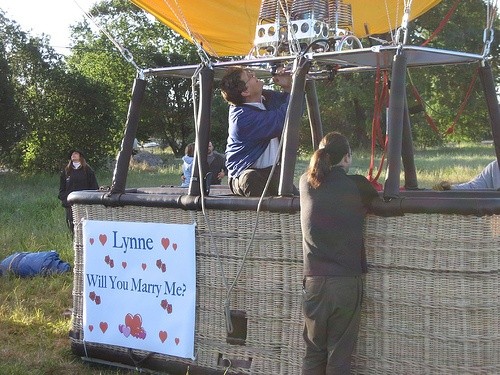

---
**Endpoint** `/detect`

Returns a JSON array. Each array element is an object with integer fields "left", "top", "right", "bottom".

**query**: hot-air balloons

[{"left": 63, "top": 0, "right": 500, "bottom": 375}]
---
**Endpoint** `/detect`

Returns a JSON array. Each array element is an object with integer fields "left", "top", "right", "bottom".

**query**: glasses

[{"left": 245, "top": 71, "right": 257, "bottom": 84}]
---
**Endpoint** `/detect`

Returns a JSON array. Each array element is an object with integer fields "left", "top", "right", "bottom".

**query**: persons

[
  {"left": 299, "top": 132, "right": 379, "bottom": 375},
  {"left": 181, "top": 139, "right": 228, "bottom": 185},
  {"left": 181, "top": 143, "right": 195, "bottom": 188},
  {"left": 431, "top": 159, "right": 500, "bottom": 191},
  {"left": 58, "top": 150, "right": 99, "bottom": 240},
  {"left": 220, "top": 66, "right": 306, "bottom": 197}
]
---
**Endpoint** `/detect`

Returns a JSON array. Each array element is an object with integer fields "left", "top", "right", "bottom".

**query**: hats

[{"left": 68, "top": 149, "right": 84, "bottom": 160}]
